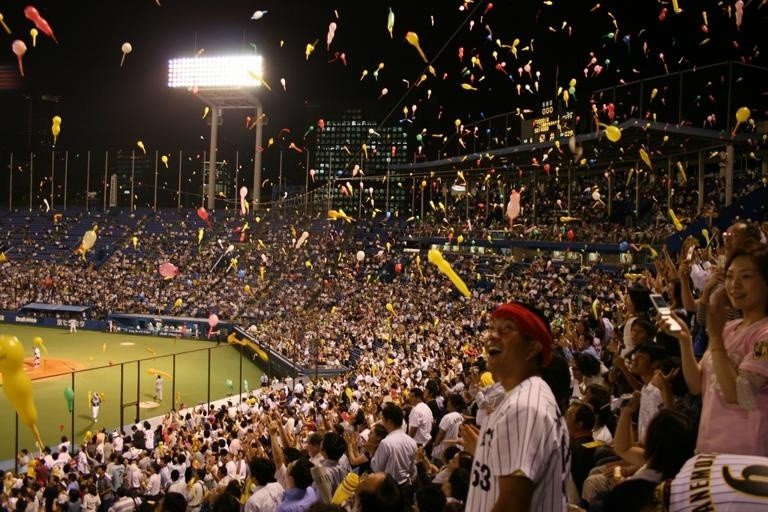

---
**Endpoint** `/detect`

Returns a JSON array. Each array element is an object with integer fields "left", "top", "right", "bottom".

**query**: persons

[{"left": 1, "top": 215, "right": 768, "bottom": 512}]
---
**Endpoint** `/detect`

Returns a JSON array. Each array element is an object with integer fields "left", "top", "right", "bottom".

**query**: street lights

[{"left": 165, "top": 52, "right": 266, "bottom": 215}]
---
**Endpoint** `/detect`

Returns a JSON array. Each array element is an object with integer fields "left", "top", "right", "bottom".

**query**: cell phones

[
  {"left": 686, "top": 247, "right": 693, "bottom": 260},
  {"left": 649, "top": 294, "right": 682, "bottom": 331},
  {"left": 417, "top": 443, "right": 423, "bottom": 448}
]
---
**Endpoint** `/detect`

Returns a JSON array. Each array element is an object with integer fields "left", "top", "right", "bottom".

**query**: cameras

[{"left": 617, "top": 395, "right": 633, "bottom": 408}]
